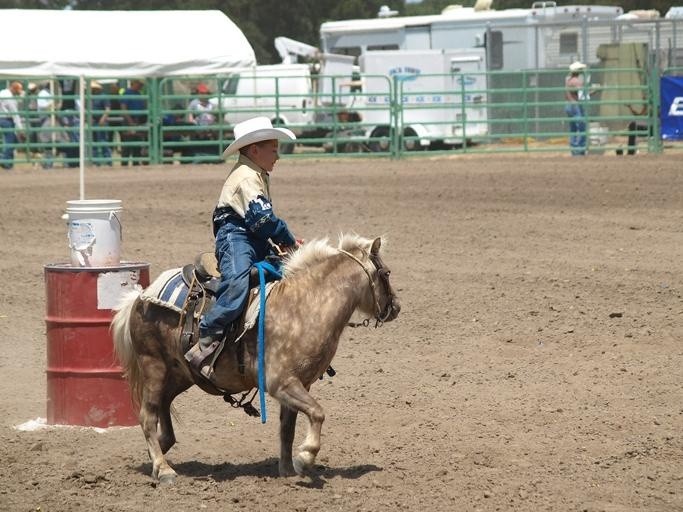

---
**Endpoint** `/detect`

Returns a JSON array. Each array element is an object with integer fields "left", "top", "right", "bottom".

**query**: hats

[
  {"left": 220, "top": 113, "right": 299, "bottom": 158},
  {"left": 569, "top": 61, "right": 588, "bottom": 74},
  {"left": 197, "top": 83, "right": 212, "bottom": 93},
  {"left": 89, "top": 80, "right": 104, "bottom": 89}
]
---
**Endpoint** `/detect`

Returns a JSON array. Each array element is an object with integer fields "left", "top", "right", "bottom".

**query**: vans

[{"left": 183, "top": 64, "right": 356, "bottom": 155}]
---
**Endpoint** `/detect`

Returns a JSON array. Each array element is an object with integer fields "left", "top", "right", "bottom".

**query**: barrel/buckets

[
  {"left": 44, "top": 265, "right": 150, "bottom": 427},
  {"left": 65, "top": 199, "right": 125, "bottom": 266}
]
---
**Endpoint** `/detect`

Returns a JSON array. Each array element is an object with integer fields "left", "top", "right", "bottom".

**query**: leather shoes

[{"left": 182, "top": 334, "right": 220, "bottom": 386}]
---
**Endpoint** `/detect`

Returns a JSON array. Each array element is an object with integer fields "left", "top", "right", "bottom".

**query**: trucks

[{"left": 356, "top": 48, "right": 490, "bottom": 150}]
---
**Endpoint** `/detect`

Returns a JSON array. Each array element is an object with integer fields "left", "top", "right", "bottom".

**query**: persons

[
  {"left": 563, "top": 60, "right": 597, "bottom": 156},
  {"left": 0, "top": 77, "right": 232, "bottom": 170},
  {"left": 196, "top": 115, "right": 307, "bottom": 374},
  {"left": 615, "top": 94, "right": 662, "bottom": 156}
]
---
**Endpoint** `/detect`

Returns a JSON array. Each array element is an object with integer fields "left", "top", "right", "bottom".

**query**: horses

[{"left": 106, "top": 229, "right": 401, "bottom": 487}]
